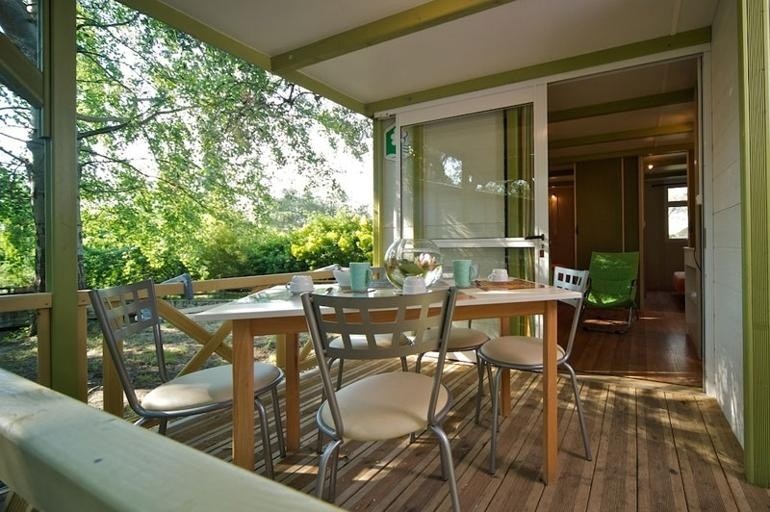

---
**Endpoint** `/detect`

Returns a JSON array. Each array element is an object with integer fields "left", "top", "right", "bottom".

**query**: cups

[
  {"left": 350, "top": 262, "right": 370, "bottom": 291},
  {"left": 402, "top": 275, "right": 425, "bottom": 293},
  {"left": 452, "top": 259, "right": 476, "bottom": 287},
  {"left": 487, "top": 270, "right": 509, "bottom": 280},
  {"left": 286, "top": 274, "right": 313, "bottom": 295}
]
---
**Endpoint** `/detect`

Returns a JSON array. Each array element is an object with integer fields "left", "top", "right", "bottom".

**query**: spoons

[{"left": 300, "top": 287, "right": 333, "bottom": 297}]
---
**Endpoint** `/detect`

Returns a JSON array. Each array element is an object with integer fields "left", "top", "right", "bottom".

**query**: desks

[{"left": 193, "top": 275, "right": 583, "bottom": 487}]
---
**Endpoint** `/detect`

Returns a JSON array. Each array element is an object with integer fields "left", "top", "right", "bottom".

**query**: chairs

[
  {"left": 415, "top": 264, "right": 501, "bottom": 432},
  {"left": 479, "top": 261, "right": 591, "bottom": 476},
  {"left": 89, "top": 275, "right": 287, "bottom": 483},
  {"left": 581, "top": 251, "right": 639, "bottom": 332},
  {"left": 303, "top": 265, "right": 461, "bottom": 511}
]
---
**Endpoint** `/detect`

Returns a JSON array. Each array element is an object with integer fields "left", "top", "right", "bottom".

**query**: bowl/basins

[{"left": 333, "top": 271, "right": 351, "bottom": 289}]
